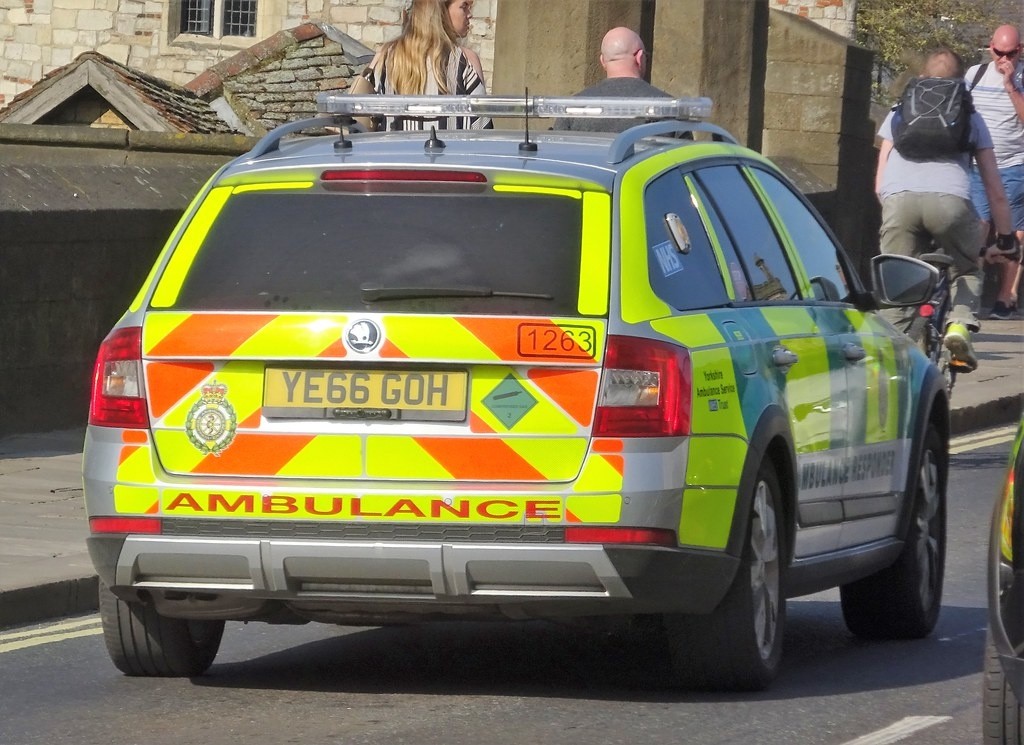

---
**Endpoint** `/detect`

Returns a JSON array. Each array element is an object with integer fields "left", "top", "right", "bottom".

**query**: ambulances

[{"left": 79, "top": 92, "right": 952, "bottom": 703}]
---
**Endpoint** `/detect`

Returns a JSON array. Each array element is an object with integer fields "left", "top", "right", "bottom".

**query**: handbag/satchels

[{"left": 326, "top": 49, "right": 384, "bottom": 137}]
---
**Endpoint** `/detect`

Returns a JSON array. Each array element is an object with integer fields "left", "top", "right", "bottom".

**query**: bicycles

[{"left": 908, "top": 229, "right": 1022, "bottom": 393}]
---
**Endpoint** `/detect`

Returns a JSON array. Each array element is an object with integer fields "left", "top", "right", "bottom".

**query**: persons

[
  {"left": 554, "top": 26, "right": 697, "bottom": 142},
  {"left": 960, "top": 26, "right": 1023, "bottom": 317},
  {"left": 873, "top": 44, "right": 1012, "bottom": 371},
  {"left": 340, "top": 1, "right": 494, "bottom": 130}
]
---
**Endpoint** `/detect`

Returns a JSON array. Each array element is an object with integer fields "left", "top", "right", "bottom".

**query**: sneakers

[
  {"left": 943, "top": 323, "right": 978, "bottom": 367},
  {"left": 989, "top": 296, "right": 1020, "bottom": 320}
]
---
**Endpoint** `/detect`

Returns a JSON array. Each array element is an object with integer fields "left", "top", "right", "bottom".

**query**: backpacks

[{"left": 891, "top": 75, "right": 974, "bottom": 160}]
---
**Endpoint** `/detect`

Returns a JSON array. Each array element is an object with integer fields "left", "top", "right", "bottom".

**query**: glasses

[{"left": 992, "top": 46, "right": 1020, "bottom": 58}]
